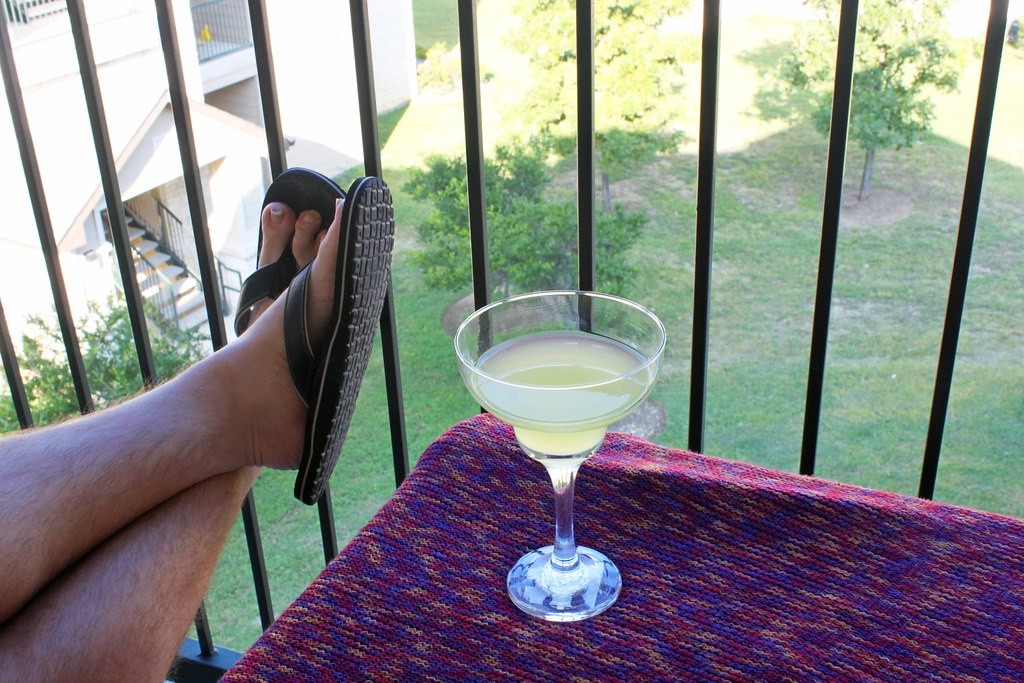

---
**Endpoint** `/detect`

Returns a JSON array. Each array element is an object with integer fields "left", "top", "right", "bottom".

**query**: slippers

[
  {"left": 287, "top": 178, "right": 395, "bottom": 507},
  {"left": 235, "top": 168, "right": 344, "bottom": 340}
]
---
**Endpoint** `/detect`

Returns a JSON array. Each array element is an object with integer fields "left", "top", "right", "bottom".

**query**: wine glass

[{"left": 454, "top": 291, "right": 666, "bottom": 619}]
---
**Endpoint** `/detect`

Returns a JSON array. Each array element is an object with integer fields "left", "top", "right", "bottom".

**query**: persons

[{"left": 1, "top": 166, "right": 398, "bottom": 683}]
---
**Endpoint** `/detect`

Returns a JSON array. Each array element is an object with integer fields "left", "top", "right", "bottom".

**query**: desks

[{"left": 218, "top": 412, "right": 1024, "bottom": 683}]
